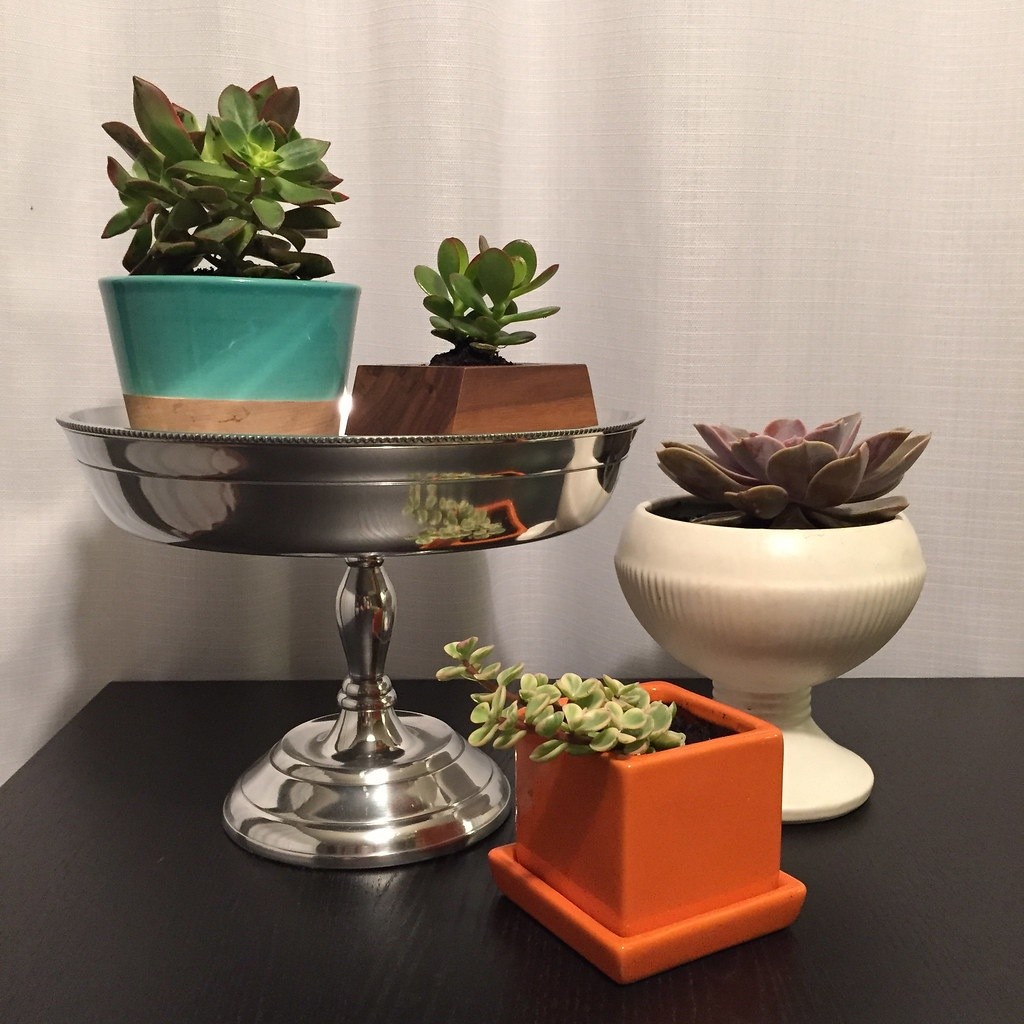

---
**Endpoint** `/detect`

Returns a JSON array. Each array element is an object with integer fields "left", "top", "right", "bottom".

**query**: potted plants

[
  {"left": 345, "top": 228, "right": 601, "bottom": 435},
  {"left": 439, "top": 635, "right": 806, "bottom": 984},
  {"left": 102, "top": 74, "right": 362, "bottom": 405},
  {"left": 400, "top": 484, "right": 528, "bottom": 551},
  {"left": 615, "top": 413, "right": 934, "bottom": 824}
]
